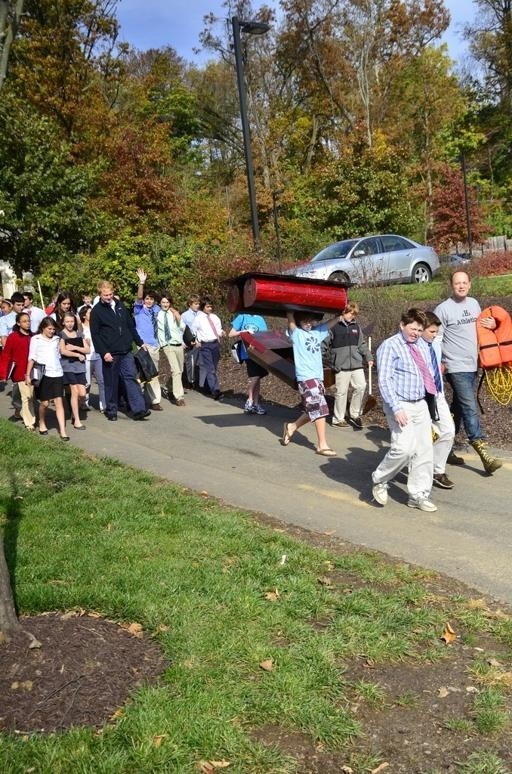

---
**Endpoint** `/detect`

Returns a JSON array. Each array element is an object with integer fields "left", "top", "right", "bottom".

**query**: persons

[
  {"left": 281, "top": 310, "right": 346, "bottom": 457},
  {"left": 320, "top": 301, "right": 374, "bottom": 429},
  {"left": 370, "top": 308, "right": 439, "bottom": 514},
  {"left": 0, "top": 265, "right": 226, "bottom": 441},
  {"left": 415, "top": 310, "right": 456, "bottom": 489},
  {"left": 227, "top": 312, "right": 269, "bottom": 415},
  {"left": 432, "top": 268, "right": 502, "bottom": 475}
]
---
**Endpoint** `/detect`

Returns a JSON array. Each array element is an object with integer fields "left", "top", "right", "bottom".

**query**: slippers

[
  {"left": 316, "top": 448, "right": 337, "bottom": 456},
  {"left": 282, "top": 422, "right": 292, "bottom": 446}
]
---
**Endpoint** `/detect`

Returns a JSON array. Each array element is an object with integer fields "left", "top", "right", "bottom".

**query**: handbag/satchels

[{"left": 231, "top": 340, "right": 245, "bottom": 364}]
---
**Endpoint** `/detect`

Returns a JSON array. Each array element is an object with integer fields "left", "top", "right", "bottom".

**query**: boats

[{"left": 244, "top": 330, "right": 337, "bottom": 391}]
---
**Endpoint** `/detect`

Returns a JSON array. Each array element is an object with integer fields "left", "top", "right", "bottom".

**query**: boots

[
  {"left": 448, "top": 451, "right": 463, "bottom": 465},
  {"left": 471, "top": 438, "right": 502, "bottom": 473}
]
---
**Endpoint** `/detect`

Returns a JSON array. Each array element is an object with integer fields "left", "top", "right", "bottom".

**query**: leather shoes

[{"left": 151, "top": 403, "right": 162, "bottom": 410}]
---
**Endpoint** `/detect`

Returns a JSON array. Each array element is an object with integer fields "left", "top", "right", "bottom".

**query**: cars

[{"left": 295, "top": 234, "right": 471, "bottom": 288}]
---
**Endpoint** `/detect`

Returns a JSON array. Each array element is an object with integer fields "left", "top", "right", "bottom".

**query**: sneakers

[
  {"left": 433, "top": 473, "right": 455, "bottom": 488},
  {"left": 244, "top": 399, "right": 267, "bottom": 415},
  {"left": 333, "top": 421, "right": 350, "bottom": 428},
  {"left": 14, "top": 409, "right": 86, "bottom": 441},
  {"left": 349, "top": 417, "right": 365, "bottom": 427},
  {"left": 214, "top": 392, "right": 224, "bottom": 400},
  {"left": 177, "top": 399, "right": 186, "bottom": 406},
  {"left": 82, "top": 400, "right": 89, "bottom": 410},
  {"left": 133, "top": 410, "right": 151, "bottom": 420},
  {"left": 110, "top": 414, "right": 117, "bottom": 421},
  {"left": 373, "top": 481, "right": 390, "bottom": 504},
  {"left": 408, "top": 497, "right": 437, "bottom": 511}
]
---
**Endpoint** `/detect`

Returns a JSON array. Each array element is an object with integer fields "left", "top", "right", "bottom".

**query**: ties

[
  {"left": 207, "top": 314, "right": 220, "bottom": 343},
  {"left": 149, "top": 309, "right": 158, "bottom": 338},
  {"left": 164, "top": 311, "right": 172, "bottom": 341},
  {"left": 427, "top": 343, "right": 442, "bottom": 393},
  {"left": 406, "top": 342, "right": 437, "bottom": 395}
]
